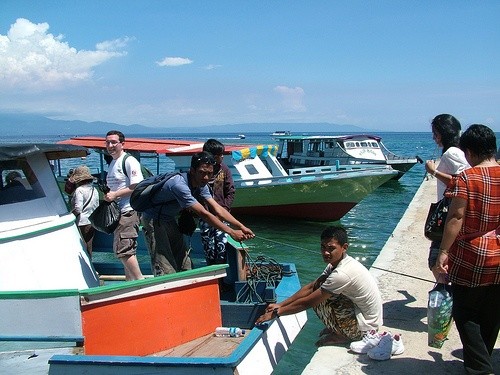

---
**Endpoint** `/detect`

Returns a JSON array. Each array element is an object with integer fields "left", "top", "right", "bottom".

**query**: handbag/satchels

[
  {"left": 130, "top": 171, "right": 186, "bottom": 213},
  {"left": 425, "top": 175, "right": 459, "bottom": 241},
  {"left": 426, "top": 271, "right": 458, "bottom": 349},
  {"left": 90, "top": 184, "right": 120, "bottom": 235}
]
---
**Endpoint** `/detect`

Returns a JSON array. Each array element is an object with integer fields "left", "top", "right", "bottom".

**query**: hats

[{"left": 69, "top": 165, "right": 93, "bottom": 183}]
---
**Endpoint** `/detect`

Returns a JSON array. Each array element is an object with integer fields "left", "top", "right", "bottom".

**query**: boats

[
  {"left": 166, "top": 143, "right": 399, "bottom": 226},
  {"left": 269, "top": 130, "right": 293, "bottom": 136},
  {"left": 48, "top": 135, "right": 248, "bottom": 274},
  {"left": 274, "top": 134, "right": 424, "bottom": 183},
  {"left": 1, "top": 141, "right": 309, "bottom": 375}
]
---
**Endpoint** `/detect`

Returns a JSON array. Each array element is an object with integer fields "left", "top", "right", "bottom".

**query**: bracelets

[
  {"left": 432, "top": 169, "right": 439, "bottom": 177},
  {"left": 273, "top": 307, "right": 280, "bottom": 318}
]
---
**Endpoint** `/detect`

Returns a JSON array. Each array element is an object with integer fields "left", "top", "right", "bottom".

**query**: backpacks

[{"left": 121, "top": 153, "right": 155, "bottom": 181}]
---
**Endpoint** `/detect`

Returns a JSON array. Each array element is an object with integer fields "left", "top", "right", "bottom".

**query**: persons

[
  {"left": 4, "top": 171, "right": 23, "bottom": 186},
  {"left": 186, "top": 138, "right": 236, "bottom": 292},
  {"left": 435, "top": 121, "right": 500, "bottom": 375},
  {"left": 141, "top": 150, "right": 256, "bottom": 278},
  {"left": 424, "top": 112, "right": 472, "bottom": 285},
  {"left": 254, "top": 225, "right": 384, "bottom": 347},
  {"left": 70, "top": 163, "right": 100, "bottom": 259},
  {"left": 103, "top": 129, "right": 146, "bottom": 283}
]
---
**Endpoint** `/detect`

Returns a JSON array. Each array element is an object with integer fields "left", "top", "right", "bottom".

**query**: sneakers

[
  {"left": 368, "top": 332, "right": 406, "bottom": 361},
  {"left": 350, "top": 329, "right": 387, "bottom": 353}
]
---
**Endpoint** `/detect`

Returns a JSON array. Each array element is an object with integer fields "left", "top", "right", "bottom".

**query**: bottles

[{"left": 215, "top": 327, "right": 245, "bottom": 337}]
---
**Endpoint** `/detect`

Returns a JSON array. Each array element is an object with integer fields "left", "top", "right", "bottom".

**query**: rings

[{"left": 436, "top": 266, "right": 439, "bottom": 269}]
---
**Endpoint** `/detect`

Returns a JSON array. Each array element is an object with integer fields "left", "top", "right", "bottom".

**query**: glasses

[{"left": 192, "top": 156, "right": 217, "bottom": 167}]
